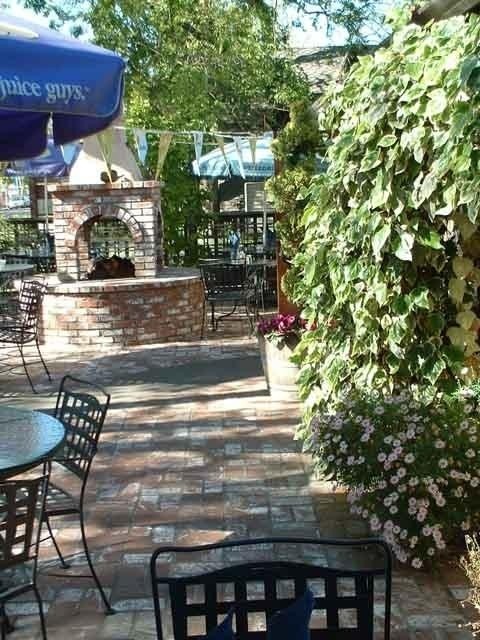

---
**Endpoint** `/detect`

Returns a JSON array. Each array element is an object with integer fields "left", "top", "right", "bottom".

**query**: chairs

[
  {"left": 198, "top": 257, "right": 259, "bottom": 340},
  {"left": 150, "top": 535, "right": 393, "bottom": 640},
  {"left": 0, "top": 375, "right": 117, "bottom": 640},
  {"left": 0, "top": 279, "right": 53, "bottom": 394}
]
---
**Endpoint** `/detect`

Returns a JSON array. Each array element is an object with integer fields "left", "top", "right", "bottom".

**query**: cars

[{"left": 9, "top": 197, "right": 30, "bottom": 208}]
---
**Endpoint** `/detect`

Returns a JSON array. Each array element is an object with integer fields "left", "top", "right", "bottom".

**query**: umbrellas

[
  {"left": 0, "top": 10, "right": 126, "bottom": 164},
  {"left": 190, "top": 130, "right": 330, "bottom": 279},
  {"left": 0, "top": 133, "right": 83, "bottom": 241}
]
